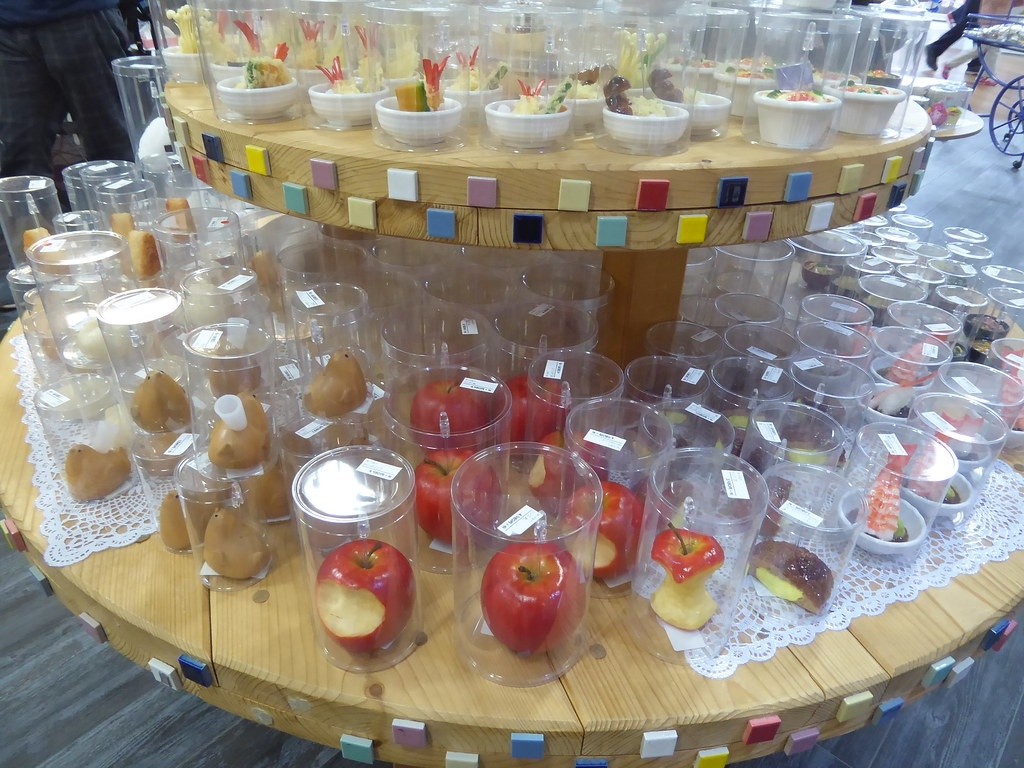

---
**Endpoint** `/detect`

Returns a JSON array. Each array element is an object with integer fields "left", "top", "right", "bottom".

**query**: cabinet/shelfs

[{"left": 0, "top": 76, "right": 1024, "bottom": 768}]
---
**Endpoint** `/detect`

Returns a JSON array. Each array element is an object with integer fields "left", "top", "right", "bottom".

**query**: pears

[{"left": 65, "top": 341, "right": 369, "bottom": 580}]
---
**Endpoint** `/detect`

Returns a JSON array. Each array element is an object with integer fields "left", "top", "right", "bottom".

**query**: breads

[{"left": 20, "top": 196, "right": 280, "bottom": 304}]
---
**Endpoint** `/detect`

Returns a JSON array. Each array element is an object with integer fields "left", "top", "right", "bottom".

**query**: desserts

[{"left": 164, "top": 5, "right": 910, "bottom": 148}]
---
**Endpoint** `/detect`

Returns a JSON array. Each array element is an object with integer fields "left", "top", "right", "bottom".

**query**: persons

[
  {"left": 925, "top": 0, "right": 1013, "bottom": 86},
  {"left": 0, "top": 0, "right": 156, "bottom": 308}
]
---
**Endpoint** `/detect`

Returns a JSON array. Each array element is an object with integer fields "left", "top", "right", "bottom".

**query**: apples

[{"left": 312, "top": 376, "right": 725, "bottom": 658}]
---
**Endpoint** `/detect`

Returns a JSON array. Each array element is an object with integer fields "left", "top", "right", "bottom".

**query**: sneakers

[
  {"left": 925, "top": 45, "right": 939, "bottom": 71},
  {"left": 942, "top": 64, "right": 950, "bottom": 79},
  {"left": 975, "top": 76, "right": 998, "bottom": 87},
  {"left": 965, "top": 68, "right": 986, "bottom": 74}
]
